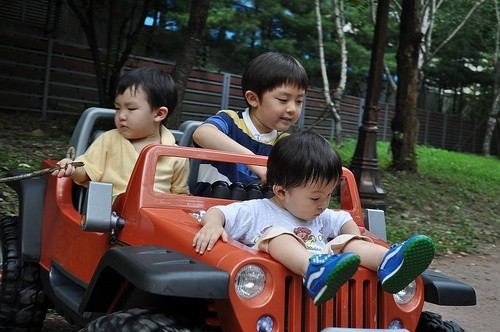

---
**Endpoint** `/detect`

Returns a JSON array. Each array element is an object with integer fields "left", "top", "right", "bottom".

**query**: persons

[
  {"left": 52, "top": 65, "right": 194, "bottom": 206},
  {"left": 190, "top": 128, "right": 437, "bottom": 306},
  {"left": 188, "top": 51, "right": 310, "bottom": 200}
]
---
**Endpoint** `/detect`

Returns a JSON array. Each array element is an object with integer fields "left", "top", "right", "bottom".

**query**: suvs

[{"left": 0, "top": 108, "right": 478, "bottom": 332}]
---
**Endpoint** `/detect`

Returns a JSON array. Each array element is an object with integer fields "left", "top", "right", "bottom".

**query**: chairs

[{"left": 60, "top": 105, "right": 118, "bottom": 214}]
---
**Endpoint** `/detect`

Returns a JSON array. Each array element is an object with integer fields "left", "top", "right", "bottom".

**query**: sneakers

[
  {"left": 376, "top": 234, "right": 435, "bottom": 294},
  {"left": 301, "top": 251, "right": 362, "bottom": 305}
]
